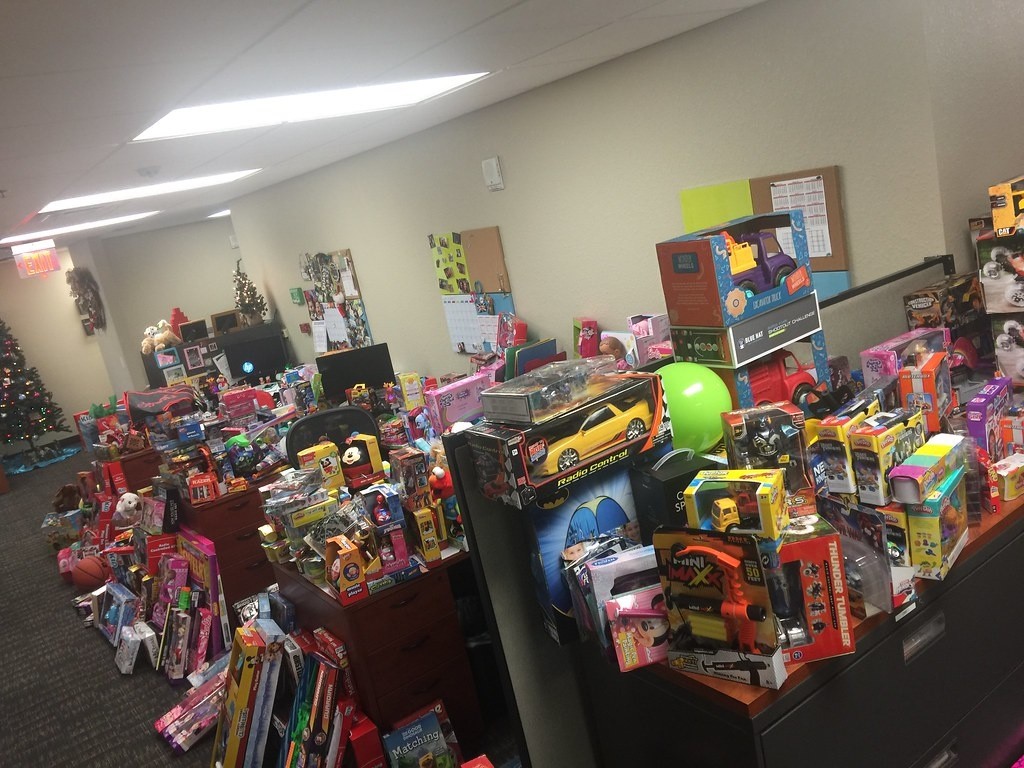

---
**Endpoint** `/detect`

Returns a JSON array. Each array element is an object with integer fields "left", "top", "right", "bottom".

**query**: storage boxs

[
  {"left": 670, "top": 289, "right": 823, "bottom": 371},
  {"left": 655, "top": 208, "right": 816, "bottom": 327},
  {"left": 903, "top": 270, "right": 989, "bottom": 339},
  {"left": 974, "top": 228, "right": 1023, "bottom": 314},
  {"left": 41, "top": 314, "right": 1024, "bottom": 768},
  {"left": 989, "top": 175, "right": 1024, "bottom": 237},
  {"left": 968, "top": 215, "right": 994, "bottom": 257}
]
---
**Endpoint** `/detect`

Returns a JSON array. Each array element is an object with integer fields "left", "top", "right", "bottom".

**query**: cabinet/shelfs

[
  {"left": 120, "top": 445, "right": 162, "bottom": 494},
  {"left": 445, "top": 256, "right": 1024, "bottom": 768},
  {"left": 179, "top": 471, "right": 288, "bottom": 629},
  {"left": 270, "top": 554, "right": 481, "bottom": 734}
]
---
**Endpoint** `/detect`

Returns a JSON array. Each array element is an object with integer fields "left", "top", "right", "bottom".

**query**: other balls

[{"left": 653, "top": 362, "right": 733, "bottom": 455}]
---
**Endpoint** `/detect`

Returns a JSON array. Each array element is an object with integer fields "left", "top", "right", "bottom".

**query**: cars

[{"left": 524, "top": 394, "right": 654, "bottom": 476}]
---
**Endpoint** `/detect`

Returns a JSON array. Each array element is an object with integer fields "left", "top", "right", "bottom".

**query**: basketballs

[{"left": 72, "top": 555, "right": 109, "bottom": 590}]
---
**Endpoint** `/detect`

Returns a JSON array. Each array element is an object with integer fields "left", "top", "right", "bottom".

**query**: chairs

[{"left": 287, "top": 407, "right": 382, "bottom": 469}]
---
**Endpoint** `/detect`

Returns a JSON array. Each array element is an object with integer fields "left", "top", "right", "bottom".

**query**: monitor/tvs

[
  {"left": 221, "top": 333, "right": 288, "bottom": 379},
  {"left": 313, "top": 341, "right": 397, "bottom": 400}
]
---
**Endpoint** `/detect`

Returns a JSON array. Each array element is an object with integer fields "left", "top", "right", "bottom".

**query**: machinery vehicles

[
  {"left": 710, "top": 491, "right": 760, "bottom": 534},
  {"left": 707, "top": 231, "right": 798, "bottom": 297},
  {"left": 748, "top": 349, "right": 817, "bottom": 405}
]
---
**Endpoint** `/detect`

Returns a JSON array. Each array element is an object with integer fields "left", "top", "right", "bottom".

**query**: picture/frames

[
  {"left": 211, "top": 309, "right": 242, "bottom": 335},
  {"left": 178, "top": 319, "right": 209, "bottom": 345},
  {"left": 166, "top": 378, "right": 188, "bottom": 388},
  {"left": 162, "top": 364, "right": 188, "bottom": 382},
  {"left": 188, "top": 372, "right": 208, "bottom": 389},
  {"left": 155, "top": 348, "right": 181, "bottom": 369},
  {"left": 184, "top": 346, "right": 205, "bottom": 370}
]
